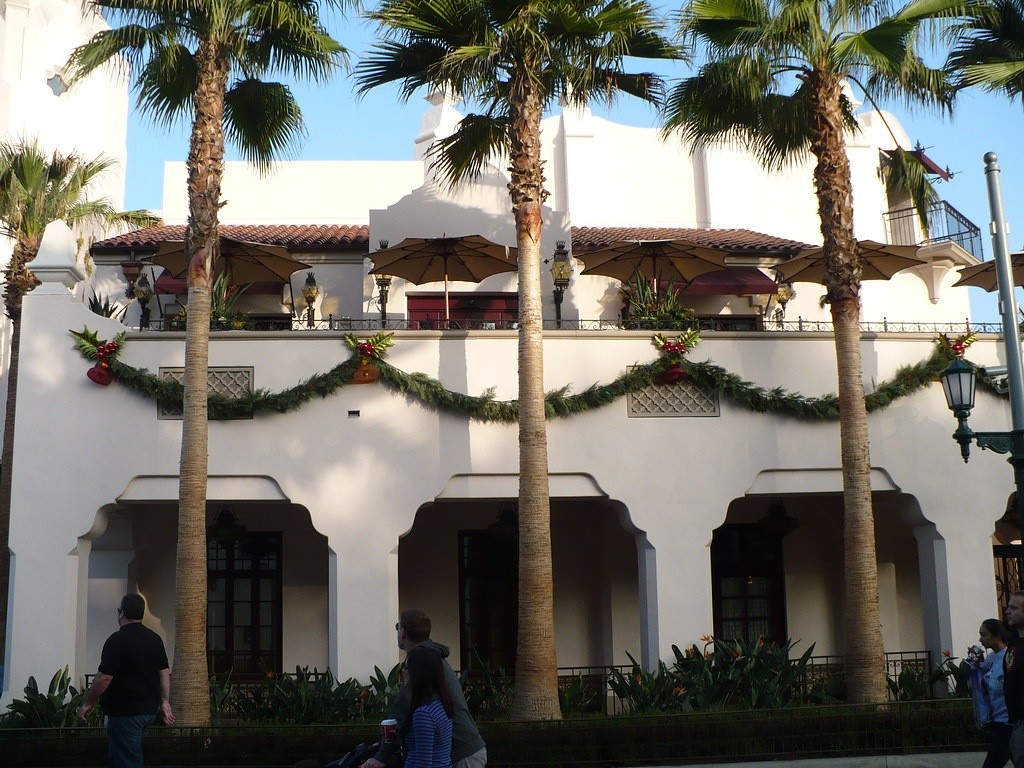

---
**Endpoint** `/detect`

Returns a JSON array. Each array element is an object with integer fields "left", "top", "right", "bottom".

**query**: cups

[
  {"left": 379, "top": 719, "right": 397, "bottom": 744},
  {"left": 965, "top": 645, "right": 985, "bottom": 667}
]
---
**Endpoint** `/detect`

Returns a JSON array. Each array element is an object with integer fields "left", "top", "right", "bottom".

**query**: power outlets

[{"left": 342, "top": 316, "right": 352, "bottom": 323}]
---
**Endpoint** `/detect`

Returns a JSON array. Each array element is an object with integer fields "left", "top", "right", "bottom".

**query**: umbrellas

[
  {"left": 575, "top": 237, "right": 732, "bottom": 302},
  {"left": 365, "top": 235, "right": 519, "bottom": 329},
  {"left": 141, "top": 238, "right": 315, "bottom": 311},
  {"left": 951, "top": 250, "right": 1024, "bottom": 292},
  {"left": 769, "top": 241, "right": 924, "bottom": 286}
]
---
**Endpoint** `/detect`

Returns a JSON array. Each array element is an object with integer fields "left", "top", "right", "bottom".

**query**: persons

[
  {"left": 362, "top": 610, "right": 487, "bottom": 768},
  {"left": 80, "top": 593, "right": 176, "bottom": 768},
  {"left": 950, "top": 590, "right": 1024, "bottom": 768}
]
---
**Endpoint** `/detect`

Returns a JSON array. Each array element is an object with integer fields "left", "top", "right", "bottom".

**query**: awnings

[{"left": 880, "top": 149, "right": 950, "bottom": 182}]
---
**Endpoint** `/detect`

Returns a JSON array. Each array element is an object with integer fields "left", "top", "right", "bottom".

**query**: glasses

[
  {"left": 396, "top": 623, "right": 403, "bottom": 631},
  {"left": 118, "top": 608, "right": 123, "bottom": 612},
  {"left": 401, "top": 666, "right": 408, "bottom": 673}
]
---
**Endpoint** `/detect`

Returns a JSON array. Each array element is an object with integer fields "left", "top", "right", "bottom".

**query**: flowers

[
  {"left": 617, "top": 265, "right": 703, "bottom": 331},
  {"left": 170, "top": 274, "right": 255, "bottom": 330}
]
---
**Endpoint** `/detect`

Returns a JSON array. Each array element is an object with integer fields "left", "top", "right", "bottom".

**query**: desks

[{"left": 689, "top": 314, "right": 763, "bottom": 331}]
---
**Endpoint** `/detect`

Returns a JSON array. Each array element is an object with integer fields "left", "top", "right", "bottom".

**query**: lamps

[
  {"left": 301, "top": 271, "right": 319, "bottom": 327},
  {"left": 544, "top": 241, "right": 573, "bottom": 328},
  {"left": 371, "top": 240, "right": 393, "bottom": 328},
  {"left": 774, "top": 271, "right": 794, "bottom": 311},
  {"left": 134, "top": 274, "right": 152, "bottom": 333}
]
